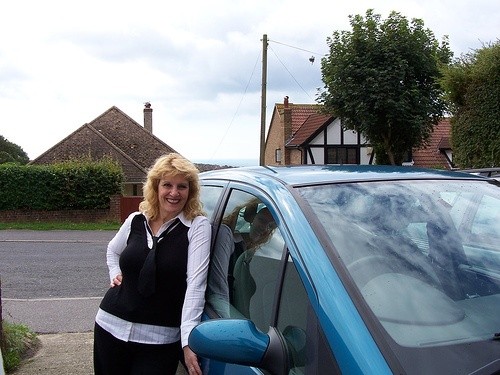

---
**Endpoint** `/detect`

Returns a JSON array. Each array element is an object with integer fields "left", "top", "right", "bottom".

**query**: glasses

[{"left": 249, "top": 221, "right": 269, "bottom": 235}]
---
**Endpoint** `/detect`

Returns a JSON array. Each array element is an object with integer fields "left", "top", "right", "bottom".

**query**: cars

[{"left": 181, "top": 162, "right": 500, "bottom": 375}]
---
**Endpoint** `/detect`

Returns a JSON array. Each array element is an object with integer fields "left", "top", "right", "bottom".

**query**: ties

[{"left": 137, "top": 219, "right": 182, "bottom": 297}]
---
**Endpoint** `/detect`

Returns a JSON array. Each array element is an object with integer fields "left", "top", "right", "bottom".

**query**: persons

[
  {"left": 201, "top": 197, "right": 279, "bottom": 375},
  {"left": 93, "top": 153, "right": 212, "bottom": 375}
]
